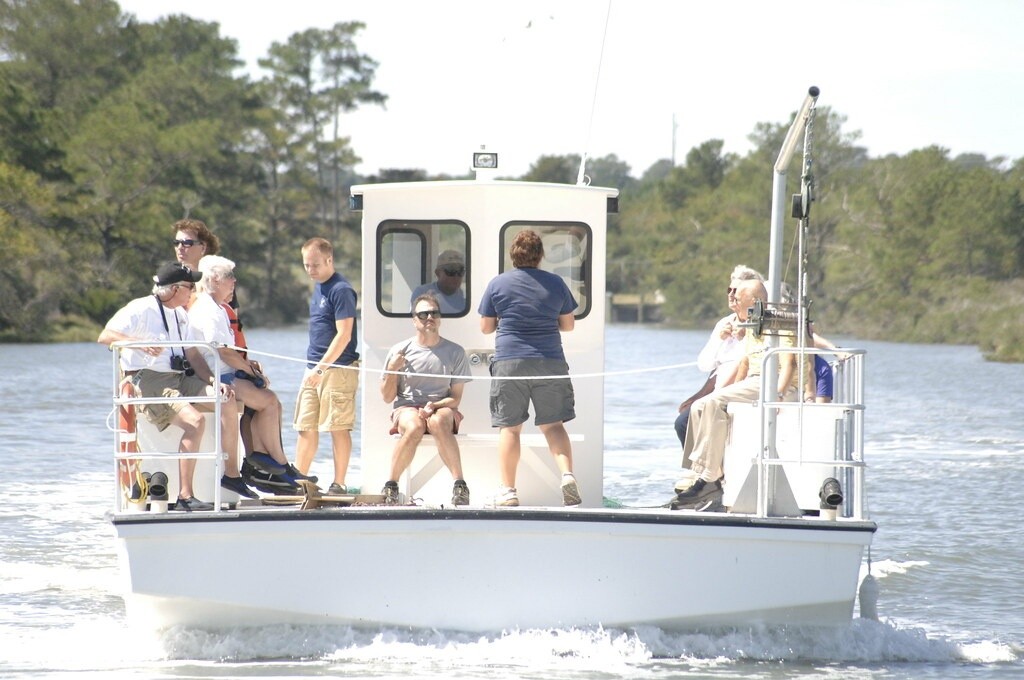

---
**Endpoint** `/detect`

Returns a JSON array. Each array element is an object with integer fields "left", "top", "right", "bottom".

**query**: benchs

[
  {"left": 386, "top": 432, "right": 585, "bottom": 507},
  {"left": 724, "top": 400, "right": 854, "bottom": 514},
  {"left": 137, "top": 411, "right": 241, "bottom": 509}
]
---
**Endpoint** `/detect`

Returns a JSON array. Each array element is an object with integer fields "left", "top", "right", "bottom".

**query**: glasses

[
  {"left": 444, "top": 268, "right": 465, "bottom": 277},
  {"left": 173, "top": 240, "right": 200, "bottom": 247},
  {"left": 728, "top": 287, "right": 737, "bottom": 294},
  {"left": 417, "top": 311, "right": 440, "bottom": 320},
  {"left": 177, "top": 284, "right": 194, "bottom": 290}
]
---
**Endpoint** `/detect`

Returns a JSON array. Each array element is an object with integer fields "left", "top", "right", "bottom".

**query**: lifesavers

[{"left": 118, "top": 381, "right": 137, "bottom": 490}]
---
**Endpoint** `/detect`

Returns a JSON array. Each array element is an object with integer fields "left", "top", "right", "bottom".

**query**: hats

[
  {"left": 154, "top": 261, "right": 202, "bottom": 285},
  {"left": 437, "top": 250, "right": 464, "bottom": 266}
]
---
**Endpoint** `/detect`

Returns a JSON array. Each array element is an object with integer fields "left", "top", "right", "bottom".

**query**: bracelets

[{"left": 776, "top": 391, "right": 783, "bottom": 398}]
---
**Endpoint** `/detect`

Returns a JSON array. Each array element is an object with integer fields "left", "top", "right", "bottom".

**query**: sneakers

[
  {"left": 382, "top": 481, "right": 399, "bottom": 506},
  {"left": 280, "top": 462, "right": 301, "bottom": 487},
  {"left": 452, "top": 479, "right": 469, "bottom": 505},
  {"left": 221, "top": 474, "right": 259, "bottom": 499},
  {"left": 248, "top": 452, "right": 286, "bottom": 474},
  {"left": 676, "top": 479, "right": 723, "bottom": 502},
  {"left": 174, "top": 496, "right": 213, "bottom": 510}
]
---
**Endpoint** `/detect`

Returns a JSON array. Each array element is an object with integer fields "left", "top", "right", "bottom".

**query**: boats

[{"left": 110, "top": 82, "right": 875, "bottom": 632}]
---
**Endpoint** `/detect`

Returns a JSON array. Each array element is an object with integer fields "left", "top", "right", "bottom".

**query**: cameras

[{"left": 170, "top": 355, "right": 191, "bottom": 371}]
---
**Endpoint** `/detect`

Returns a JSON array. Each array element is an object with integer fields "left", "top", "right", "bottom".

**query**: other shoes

[
  {"left": 325, "top": 482, "right": 347, "bottom": 493},
  {"left": 240, "top": 458, "right": 254, "bottom": 476},
  {"left": 675, "top": 472, "right": 702, "bottom": 488},
  {"left": 561, "top": 472, "right": 581, "bottom": 506},
  {"left": 291, "top": 464, "right": 318, "bottom": 483},
  {"left": 250, "top": 468, "right": 290, "bottom": 487},
  {"left": 500, "top": 487, "right": 519, "bottom": 505}
]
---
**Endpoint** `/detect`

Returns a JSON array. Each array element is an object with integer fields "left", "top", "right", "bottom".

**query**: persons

[
  {"left": 478, "top": 229, "right": 582, "bottom": 505},
  {"left": 96, "top": 220, "right": 317, "bottom": 512},
  {"left": 292, "top": 238, "right": 362, "bottom": 496},
  {"left": 408, "top": 249, "right": 467, "bottom": 315},
  {"left": 673, "top": 265, "right": 849, "bottom": 503},
  {"left": 380, "top": 294, "right": 472, "bottom": 505}
]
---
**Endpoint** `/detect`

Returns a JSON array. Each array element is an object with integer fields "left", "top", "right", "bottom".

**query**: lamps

[{"left": 472, "top": 151, "right": 500, "bottom": 179}]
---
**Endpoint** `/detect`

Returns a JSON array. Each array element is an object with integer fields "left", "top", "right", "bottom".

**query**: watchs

[{"left": 313, "top": 366, "right": 323, "bottom": 376}]
